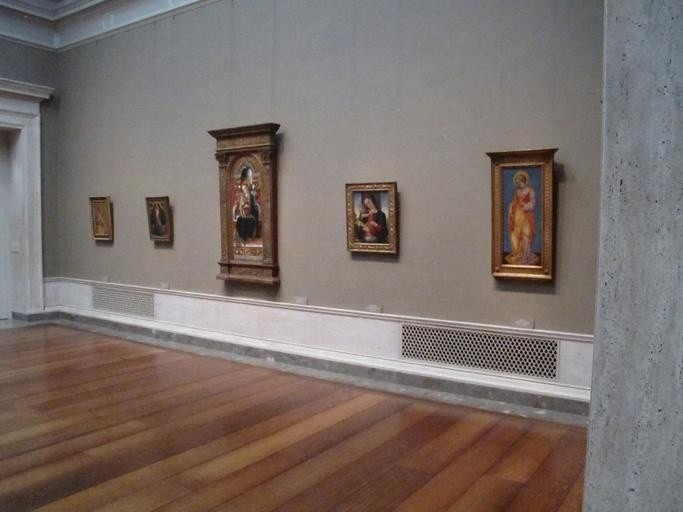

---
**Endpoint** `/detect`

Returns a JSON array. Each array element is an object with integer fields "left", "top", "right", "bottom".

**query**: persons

[
  {"left": 149, "top": 200, "right": 165, "bottom": 235},
  {"left": 353, "top": 191, "right": 388, "bottom": 244},
  {"left": 93, "top": 205, "right": 104, "bottom": 234},
  {"left": 504, "top": 170, "right": 537, "bottom": 264},
  {"left": 232, "top": 168, "right": 259, "bottom": 244}
]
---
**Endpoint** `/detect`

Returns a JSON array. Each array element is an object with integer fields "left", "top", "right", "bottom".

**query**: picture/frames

[
  {"left": 345, "top": 181, "right": 398, "bottom": 255},
  {"left": 486, "top": 148, "right": 557, "bottom": 281},
  {"left": 145, "top": 196, "right": 173, "bottom": 242},
  {"left": 89, "top": 196, "right": 113, "bottom": 242}
]
